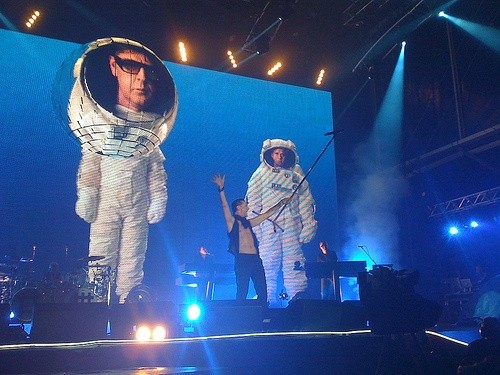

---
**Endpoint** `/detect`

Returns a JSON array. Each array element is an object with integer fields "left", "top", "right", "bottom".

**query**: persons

[
  {"left": 75, "top": 144, "right": 168, "bottom": 305},
  {"left": 108, "top": 43, "right": 163, "bottom": 128},
  {"left": 457, "top": 341, "right": 486, "bottom": 375},
  {"left": 469, "top": 319, "right": 499, "bottom": 375},
  {"left": 246, "top": 137, "right": 318, "bottom": 306},
  {"left": 319, "top": 242, "right": 342, "bottom": 300},
  {"left": 215, "top": 176, "right": 291, "bottom": 306},
  {"left": 198, "top": 244, "right": 212, "bottom": 300}
]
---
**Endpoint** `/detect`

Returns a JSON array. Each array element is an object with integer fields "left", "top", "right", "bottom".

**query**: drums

[
  {"left": 10, "top": 287, "right": 37, "bottom": 322},
  {"left": 63, "top": 273, "right": 80, "bottom": 287}
]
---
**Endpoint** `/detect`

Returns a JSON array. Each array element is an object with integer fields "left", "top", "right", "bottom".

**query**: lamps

[
  {"left": 279, "top": 8, "right": 292, "bottom": 22},
  {"left": 351, "top": 21, "right": 360, "bottom": 33},
  {"left": 240, "top": 32, "right": 271, "bottom": 55},
  {"left": 367, "top": 64, "right": 375, "bottom": 80}
]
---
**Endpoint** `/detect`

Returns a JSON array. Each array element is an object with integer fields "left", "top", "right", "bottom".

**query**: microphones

[
  {"left": 324, "top": 129, "right": 344, "bottom": 136},
  {"left": 358, "top": 246, "right": 363, "bottom": 247}
]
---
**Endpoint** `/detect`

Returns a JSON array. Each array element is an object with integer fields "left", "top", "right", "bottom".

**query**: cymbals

[
  {"left": 77, "top": 256, "right": 105, "bottom": 262},
  {"left": 84, "top": 264, "right": 106, "bottom": 267}
]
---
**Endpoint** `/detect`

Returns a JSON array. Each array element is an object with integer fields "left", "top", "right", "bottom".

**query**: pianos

[
  {"left": 306, "top": 261, "right": 366, "bottom": 267},
  {"left": 175, "top": 277, "right": 236, "bottom": 286},
  {"left": 306, "top": 269, "right": 367, "bottom": 278},
  {"left": 179, "top": 263, "right": 235, "bottom": 275}
]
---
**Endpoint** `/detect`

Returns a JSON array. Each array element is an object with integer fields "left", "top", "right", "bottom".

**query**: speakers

[
  {"left": 341, "top": 300, "right": 367, "bottom": 330},
  {"left": 30, "top": 301, "right": 176, "bottom": 340},
  {"left": 204, "top": 299, "right": 264, "bottom": 335},
  {"left": 284, "top": 299, "right": 341, "bottom": 330}
]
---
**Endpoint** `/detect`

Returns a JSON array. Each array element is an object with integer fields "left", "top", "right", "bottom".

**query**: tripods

[{"left": 252, "top": 134, "right": 336, "bottom": 233}]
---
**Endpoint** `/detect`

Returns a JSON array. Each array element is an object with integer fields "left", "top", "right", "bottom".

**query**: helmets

[
  {"left": 260, "top": 139, "right": 299, "bottom": 172},
  {"left": 52, "top": 36, "right": 178, "bottom": 160}
]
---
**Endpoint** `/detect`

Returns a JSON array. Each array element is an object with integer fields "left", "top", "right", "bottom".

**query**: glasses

[{"left": 114, "top": 55, "right": 160, "bottom": 82}]
